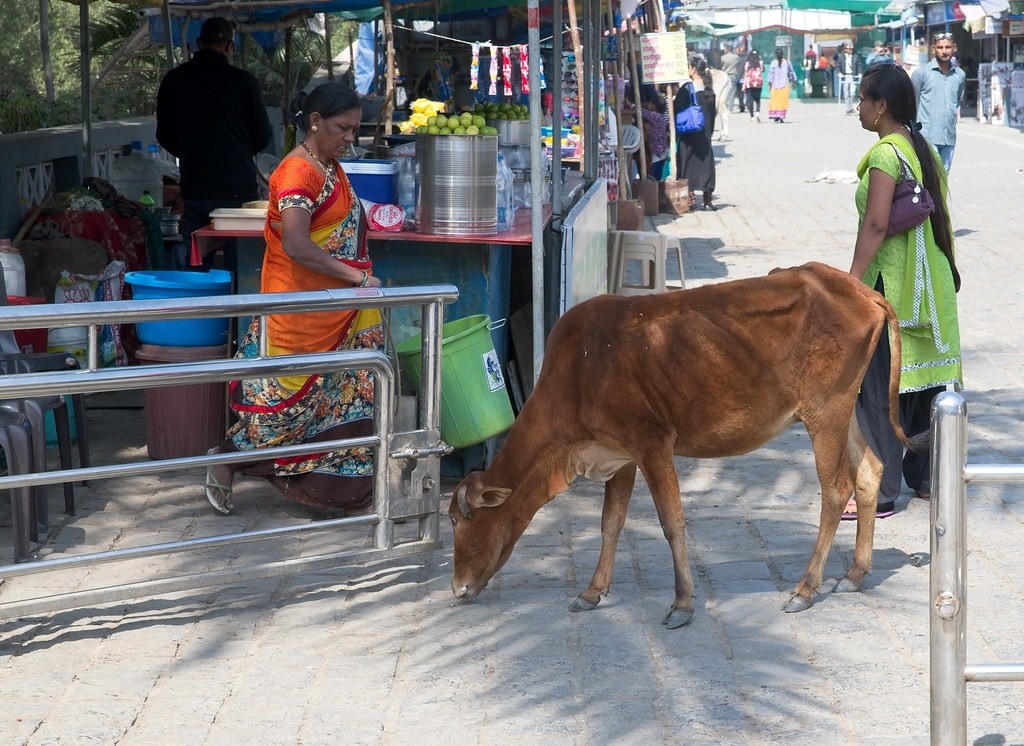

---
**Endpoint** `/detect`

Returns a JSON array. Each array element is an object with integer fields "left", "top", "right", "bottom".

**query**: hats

[
  {"left": 750, "top": 49, "right": 758, "bottom": 54},
  {"left": 846, "top": 44, "right": 853, "bottom": 48},
  {"left": 873, "top": 40, "right": 886, "bottom": 47}
]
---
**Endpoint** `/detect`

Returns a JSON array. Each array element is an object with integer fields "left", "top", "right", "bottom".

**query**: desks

[
  {"left": 161, "top": 234, "right": 183, "bottom": 271},
  {"left": 546, "top": 154, "right": 585, "bottom": 173}
]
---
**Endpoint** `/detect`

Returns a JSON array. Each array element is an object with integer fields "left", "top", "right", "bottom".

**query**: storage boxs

[
  {"left": 333, "top": 158, "right": 398, "bottom": 206},
  {"left": 7, "top": 294, "right": 50, "bottom": 353},
  {"left": 209, "top": 207, "right": 268, "bottom": 231}
]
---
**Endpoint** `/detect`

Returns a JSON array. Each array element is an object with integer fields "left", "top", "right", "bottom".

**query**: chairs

[
  {"left": 621, "top": 124, "right": 641, "bottom": 198},
  {"left": 0, "top": 261, "right": 90, "bottom": 565}
]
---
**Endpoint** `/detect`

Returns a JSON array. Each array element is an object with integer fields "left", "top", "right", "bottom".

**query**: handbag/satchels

[
  {"left": 787, "top": 60, "right": 795, "bottom": 82},
  {"left": 675, "top": 82, "right": 704, "bottom": 132},
  {"left": 884, "top": 143, "right": 935, "bottom": 235}
]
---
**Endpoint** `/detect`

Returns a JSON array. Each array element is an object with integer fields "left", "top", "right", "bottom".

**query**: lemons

[
  {"left": 416, "top": 112, "right": 499, "bottom": 135},
  {"left": 471, "top": 102, "right": 530, "bottom": 119}
]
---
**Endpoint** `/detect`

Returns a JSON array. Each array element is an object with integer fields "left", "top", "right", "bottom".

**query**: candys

[{"left": 470, "top": 43, "right": 546, "bottom": 96}]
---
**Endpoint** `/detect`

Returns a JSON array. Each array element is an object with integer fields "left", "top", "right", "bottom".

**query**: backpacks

[{"left": 748, "top": 60, "right": 761, "bottom": 88}]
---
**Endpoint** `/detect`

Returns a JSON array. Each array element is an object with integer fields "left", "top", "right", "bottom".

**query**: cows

[
  {"left": 710, "top": 69, "right": 732, "bottom": 141},
  {"left": 448, "top": 260, "right": 919, "bottom": 630}
]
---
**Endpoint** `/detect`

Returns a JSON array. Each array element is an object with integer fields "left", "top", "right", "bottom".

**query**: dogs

[{"left": 805, "top": 170, "right": 861, "bottom": 185}]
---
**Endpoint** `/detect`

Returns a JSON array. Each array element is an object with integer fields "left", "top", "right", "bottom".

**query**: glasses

[{"left": 934, "top": 32, "right": 953, "bottom": 43}]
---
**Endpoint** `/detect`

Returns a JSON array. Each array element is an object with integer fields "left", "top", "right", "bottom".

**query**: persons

[
  {"left": 623, "top": 50, "right": 717, "bottom": 211},
  {"left": 204, "top": 82, "right": 388, "bottom": 514},
  {"left": 155, "top": 17, "right": 272, "bottom": 271},
  {"left": 826, "top": 40, "right": 863, "bottom": 115},
  {"left": 910, "top": 30, "right": 965, "bottom": 171},
  {"left": 805, "top": 44, "right": 838, "bottom": 70},
  {"left": 766, "top": 47, "right": 797, "bottom": 124},
  {"left": 719, "top": 44, "right": 741, "bottom": 112},
  {"left": 735, "top": 46, "right": 749, "bottom": 112},
  {"left": 741, "top": 49, "right": 765, "bottom": 122},
  {"left": 840, "top": 62, "right": 961, "bottom": 520},
  {"left": 865, "top": 40, "right": 893, "bottom": 65}
]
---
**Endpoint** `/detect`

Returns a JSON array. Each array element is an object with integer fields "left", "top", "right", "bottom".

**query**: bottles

[
  {"left": 497, "top": 155, "right": 511, "bottom": 234},
  {"left": 0, "top": 238, "right": 27, "bottom": 297},
  {"left": 138, "top": 190, "right": 155, "bottom": 215}
]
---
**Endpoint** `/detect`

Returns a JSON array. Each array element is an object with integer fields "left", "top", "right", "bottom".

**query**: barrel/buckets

[
  {"left": 395, "top": 313, "right": 516, "bottom": 450},
  {"left": 4, "top": 295, "right": 49, "bottom": 353},
  {"left": 123, "top": 268, "right": 234, "bottom": 347},
  {"left": 134, "top": 343, "right": 229, "bottom": 461},
  {"left": 411, "top": 131, "right": 499, "bottom": 238},
  {"left": 485, "top": 119, "right": 532, "bottom": 209}
]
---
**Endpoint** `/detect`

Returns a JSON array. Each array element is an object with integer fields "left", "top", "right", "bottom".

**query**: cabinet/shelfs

[{"left": 356, "top": 120, "right": 402, "bottom": 148}]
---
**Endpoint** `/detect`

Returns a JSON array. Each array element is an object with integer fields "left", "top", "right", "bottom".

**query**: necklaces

[
  {"left": 889, "top": 127, "right": 905, "bottom": 133},
  {"left": 300, "top": 142, "right": 328, "bottom": 170}
]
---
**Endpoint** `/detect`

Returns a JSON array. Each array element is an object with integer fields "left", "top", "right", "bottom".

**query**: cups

[{"left": 148, "top": 144, "right": 159, "bottom": 159}]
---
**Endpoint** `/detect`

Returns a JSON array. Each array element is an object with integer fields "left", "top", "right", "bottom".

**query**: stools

[{"left": 607, "top": 229, "right": 685, "bottom": 296}]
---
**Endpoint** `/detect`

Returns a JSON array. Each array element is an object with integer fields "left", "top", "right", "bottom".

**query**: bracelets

[{"left": 360, "top": 271, "right": 368, "bottom": 286}]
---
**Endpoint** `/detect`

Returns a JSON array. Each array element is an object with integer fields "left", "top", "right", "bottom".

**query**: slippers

[{"left": 205, "top": 448, "right": 233, "bottom": 515}]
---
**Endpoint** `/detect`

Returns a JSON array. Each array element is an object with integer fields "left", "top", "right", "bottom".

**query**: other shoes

[
  {"left": 756, "top": 116, "right": 760, "bottom": 122},
  {"left": 841, "top": 498, "right": 894, "bottom": 519},
  {"left": 774, "top": 117, "right": 784, "bottom": 123},
  {"left": 704, "top": 202, "right": 716, "bottom": 211}
]
